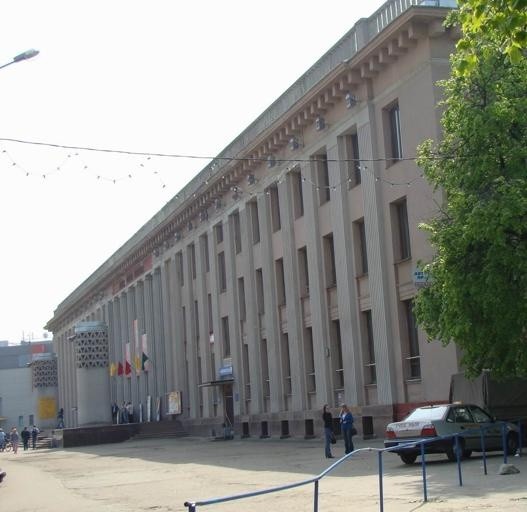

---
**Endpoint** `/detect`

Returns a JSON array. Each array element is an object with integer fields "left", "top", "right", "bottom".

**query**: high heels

[{"left": 325, "top": 455, "right": 333, "bottom": 458}]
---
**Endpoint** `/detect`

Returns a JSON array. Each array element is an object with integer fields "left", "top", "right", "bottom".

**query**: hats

[{"left": 339, "top": 403, "right": 346, "bottom": 407}]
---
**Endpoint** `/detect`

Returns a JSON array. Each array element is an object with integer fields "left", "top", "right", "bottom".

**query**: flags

[{"left": 110, "top": 352, "right": 151, "bottom": 379}]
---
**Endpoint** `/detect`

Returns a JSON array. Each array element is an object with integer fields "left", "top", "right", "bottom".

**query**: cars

[{"left": 384, "top": 401, "right": 522, "bottom": 465}]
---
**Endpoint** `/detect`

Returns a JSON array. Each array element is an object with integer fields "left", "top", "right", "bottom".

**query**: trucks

[{"left": 449, "top": 370, "right": 527, "bottom": 447}]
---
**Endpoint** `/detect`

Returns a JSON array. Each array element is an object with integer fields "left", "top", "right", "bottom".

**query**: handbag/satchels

[
  {"left": 330, "top": 432, "right": 337, "bottom": 444},
  {"left": 350, "top": 427, "right": 357, "bottom": 436}
]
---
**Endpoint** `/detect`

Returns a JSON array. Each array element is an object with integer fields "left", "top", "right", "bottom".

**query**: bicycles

[{"left": 0, "top": 438, "right": 12, "bottom": 452}]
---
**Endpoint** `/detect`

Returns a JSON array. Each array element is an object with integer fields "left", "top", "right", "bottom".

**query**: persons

[
  {"left": 112, "top": 399, "right": 134, "bottom": 425},
  {"left": 322, "top": 404, "right": 335, "bottom": 457},
  {"left": 339, "top": 403, "right": 353, "bottom": 454},
  {"left": 57, "top": 408, "right": 65, "bottom": 429},
  {"left": 0, "top": 425, "right": 40, "bottom": 453}
]
